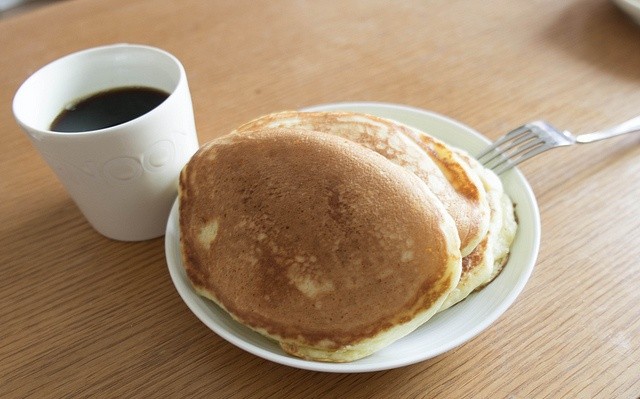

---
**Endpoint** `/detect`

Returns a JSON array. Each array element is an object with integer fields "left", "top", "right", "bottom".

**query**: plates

[{"left": 166, "top": 101, "right": 541, "bottom": 374}]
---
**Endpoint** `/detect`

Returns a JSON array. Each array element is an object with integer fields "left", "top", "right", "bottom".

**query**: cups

[{"left": 13, "top": 43, "right": 199, "bottom": 243}]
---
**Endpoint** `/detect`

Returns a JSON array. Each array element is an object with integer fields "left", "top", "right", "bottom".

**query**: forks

[{"left": 475, "top": 117, "right": 640, "bottom": 174}]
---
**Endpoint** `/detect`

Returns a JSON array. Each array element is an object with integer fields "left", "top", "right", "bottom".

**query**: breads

[{"left": 177, "top": 110, "right": 517, "bottom": 363}]
